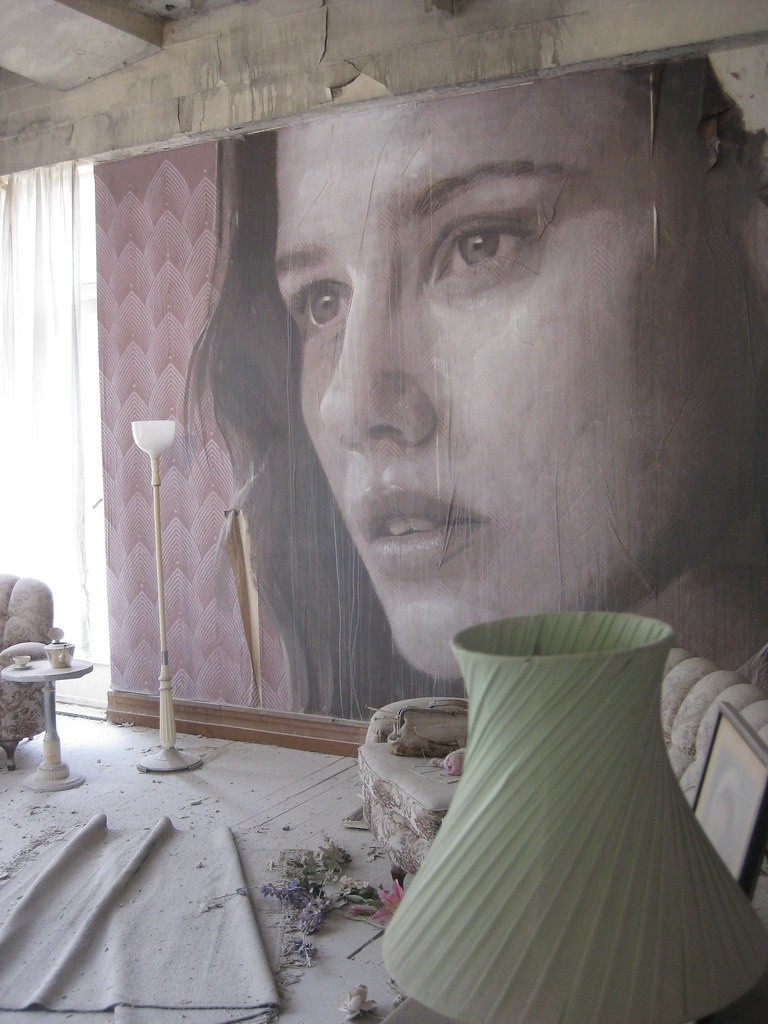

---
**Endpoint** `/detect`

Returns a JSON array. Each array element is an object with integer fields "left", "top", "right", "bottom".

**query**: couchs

[
  {"left": 355, "top": 645, "right": 768, "bottom": 922},
  {"left": 0, "top": 572, "right": 57, "bottom": 773}
]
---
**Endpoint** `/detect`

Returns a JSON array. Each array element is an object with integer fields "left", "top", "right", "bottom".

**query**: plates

[{"left": 9, "top": 666, "right": 33, "bottom": 670}]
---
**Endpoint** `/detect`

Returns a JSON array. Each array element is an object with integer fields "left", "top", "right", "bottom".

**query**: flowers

[{"left": 191, "top": 834, "right": 406, "bottom": 966}]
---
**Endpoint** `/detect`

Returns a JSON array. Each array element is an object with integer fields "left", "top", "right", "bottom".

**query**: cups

[{"left": 10, "top": 655, "right": 31, "bottom": 667}]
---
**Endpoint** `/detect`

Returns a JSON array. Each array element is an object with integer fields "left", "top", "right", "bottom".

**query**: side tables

[{"left": 1, "top": 658, "right": 94, "bottom": 791}]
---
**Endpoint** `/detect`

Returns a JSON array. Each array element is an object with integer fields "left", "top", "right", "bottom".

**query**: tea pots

[{"left": 45, "top": 627, "right": 75, "bottom": 669}]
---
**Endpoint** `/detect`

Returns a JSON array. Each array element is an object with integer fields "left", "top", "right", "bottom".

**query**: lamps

[{"left": 128, "top": 419, "right": 206, "bottom": 773}]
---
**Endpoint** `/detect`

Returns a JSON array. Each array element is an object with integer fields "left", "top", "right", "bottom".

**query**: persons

[{"left": 181, "top": 54, "right": 766, "bottom": 719}]
